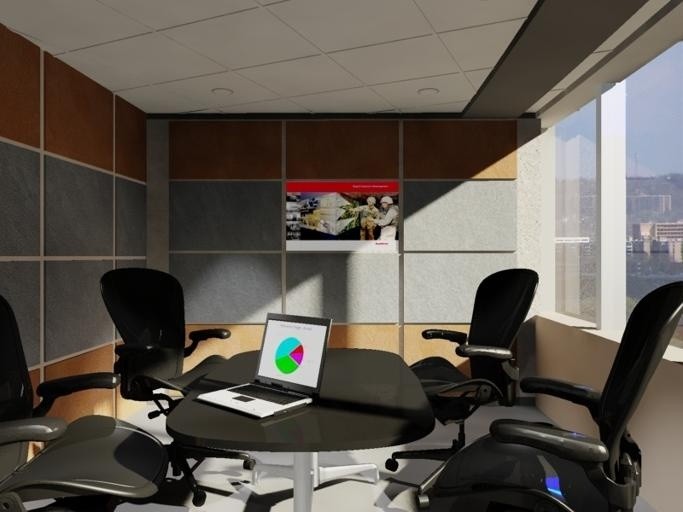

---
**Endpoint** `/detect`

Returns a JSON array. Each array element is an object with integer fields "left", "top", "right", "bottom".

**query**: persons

[
  {"left": 367, "top": 196, "right": 399, "bottom": 240},
  {"left": 347, "top": 194, "right": 379, "bottom": 241}
]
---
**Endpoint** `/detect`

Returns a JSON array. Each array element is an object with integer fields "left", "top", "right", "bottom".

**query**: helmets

[
  {"left": 380, "top": 196, "right": 393, "bottom": 204},
  {"left": 367, "top": 197, "right": 376, "bottom": 203}
]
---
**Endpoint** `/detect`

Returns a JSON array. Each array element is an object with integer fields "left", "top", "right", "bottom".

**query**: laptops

[{"left": 196, "top": 314, "right": 334, "bottom": 420}]
